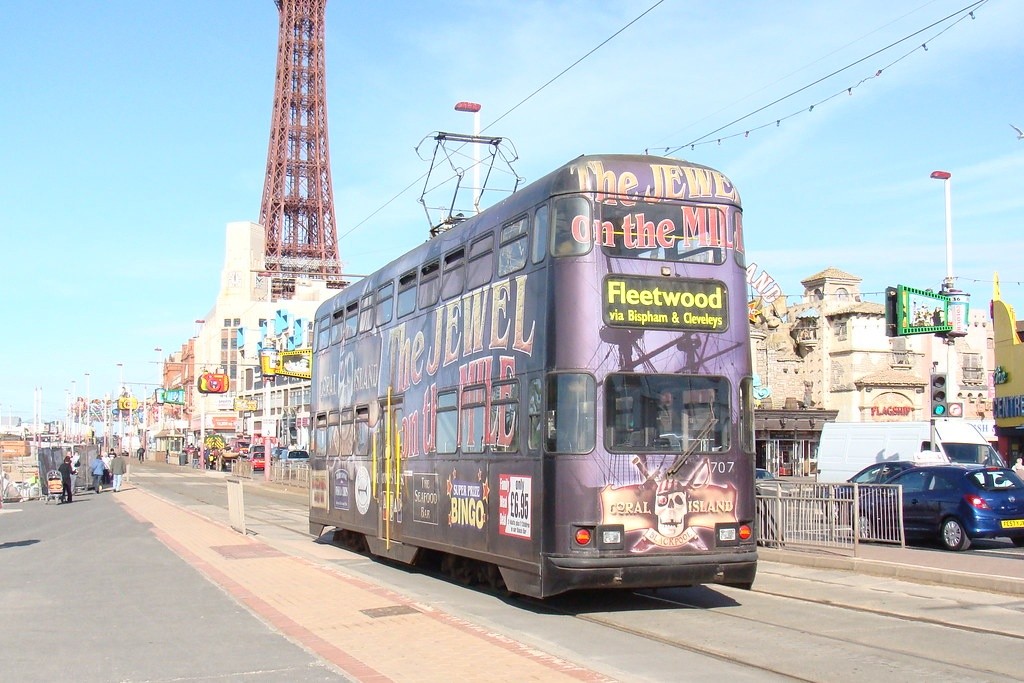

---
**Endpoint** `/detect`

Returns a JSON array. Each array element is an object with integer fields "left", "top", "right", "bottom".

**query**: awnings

[{"left": 796, "top": 309, "right": 820, "bottom": 320}]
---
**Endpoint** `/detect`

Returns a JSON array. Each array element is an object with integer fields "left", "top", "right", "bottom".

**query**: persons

[
  {"left": 58, "top": 456, "right": 76, "bottom": 503},
  {"left": 108, "top": 448, "right": 117, "bottom": 459},
  {"left": 225, "top": 444, "right": 231, "bottom": 451},
  {"left": 64, "top": 450, "right": 80, "bottom": 496},
  {"left": 204, "top": 447, "right": 211, "bottom": 469},
  {"left": 91, "top": 455, "right": 105, "bottom": 493},
  {"left": 189, "top": 443, "right": 194, "bottom": 449},
  {"left": 212, "top": 447, "right": 218, "bottom": 458},
  {"left": 102, "top": 452, "right": 111, "bottom": 485},
  {"left": 137, "top": 445, "right": 145, "bottom": 462},
  {"left": 192, "top": 448, "right": 199, "bottom": 469},
  {"left": 109, "top": 453, "right": 126, "bottom": 492},
  {"left": 1012, "top": 458, "right": 1024, "bottom": 482}
]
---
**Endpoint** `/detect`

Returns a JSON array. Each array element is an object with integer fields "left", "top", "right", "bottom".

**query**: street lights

[{"left": 930, "top": 169, "right": 956, "bottom": 292}]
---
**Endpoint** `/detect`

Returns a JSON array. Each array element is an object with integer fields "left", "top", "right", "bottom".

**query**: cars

[
  {"left": 841, "top": 462, "right": 1024, "bottom": 551},
  {"left": 755, "top": 468, "right": 789, "bottom": 513},
  {"left": 221, "top": 437, "right": 308, "bottom": 472},
  {"left": 820, "top": 461, "right": 919, "bottom": 524}
]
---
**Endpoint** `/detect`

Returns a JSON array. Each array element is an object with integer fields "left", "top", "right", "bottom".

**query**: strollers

[{"left": 45, "top": 470, "right": 65, "bottom": 505}]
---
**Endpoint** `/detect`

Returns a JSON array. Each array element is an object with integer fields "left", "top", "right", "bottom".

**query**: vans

[{"left": 815, "top": 422, "right": 1017, "bottom": 522}]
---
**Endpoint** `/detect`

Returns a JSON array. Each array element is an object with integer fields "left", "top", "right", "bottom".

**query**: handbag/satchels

[{"left": 91, "top": 472, "right": 95, "bottom": 476}]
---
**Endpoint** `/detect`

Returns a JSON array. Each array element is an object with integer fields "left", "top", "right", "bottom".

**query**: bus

[{"left": 308, "top": 130, "right": 758, "bottom": 600}]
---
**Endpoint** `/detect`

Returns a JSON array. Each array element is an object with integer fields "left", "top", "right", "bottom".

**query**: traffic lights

[{"left": 930, "top": 372, "right": 948, "bottom": 418}]
